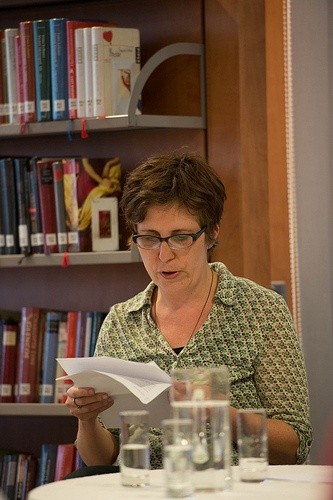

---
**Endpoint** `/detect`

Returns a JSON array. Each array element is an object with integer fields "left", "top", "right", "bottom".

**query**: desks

[{"left": 28, "top": 465, "right": 333, "bottom": 500}]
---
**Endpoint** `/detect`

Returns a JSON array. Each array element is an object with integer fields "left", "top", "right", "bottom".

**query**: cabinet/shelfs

[{"left": 0, "top": 0, "right": 210, "bottom": 419}]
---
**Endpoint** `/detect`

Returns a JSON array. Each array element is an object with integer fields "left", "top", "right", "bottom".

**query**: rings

[{"left": 74, "top": 398, "right": 80, "bottom": 408}]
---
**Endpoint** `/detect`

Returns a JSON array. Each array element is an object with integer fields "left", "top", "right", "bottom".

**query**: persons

[{"left": 63, "top": 151, "right": 313, "bottom": 479}]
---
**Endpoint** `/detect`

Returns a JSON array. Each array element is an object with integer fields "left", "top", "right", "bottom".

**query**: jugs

[{"left": 168, "top": 367, "right": 233, "bottom": 489}]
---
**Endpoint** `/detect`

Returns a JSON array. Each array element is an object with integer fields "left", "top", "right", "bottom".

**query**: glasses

[{"left": 131, "top": 224, "right": 208, "bottom": 250}]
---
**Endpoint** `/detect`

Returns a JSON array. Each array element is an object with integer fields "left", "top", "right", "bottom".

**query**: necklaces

[{"left": 154, "top": 270, "right": 215, "bottom": 370}]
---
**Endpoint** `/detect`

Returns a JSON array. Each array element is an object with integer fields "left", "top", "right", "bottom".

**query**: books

[
  {"left": 0, "top": 18, "right": 142, "bottom": 125},
  {"left": 0, "top": 443, "right": 86, "bottom": 500},
  {"left": 0, "top": 306, "right": 109, "bottom": 404},
  {"left": 0, "top": 157, "right": 130, "bottom": 255}
]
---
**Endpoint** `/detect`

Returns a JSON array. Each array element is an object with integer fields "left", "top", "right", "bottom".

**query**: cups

[
  {"left": 160, "top": 418, "right": 196, "bottom": 497},
  {"left": 236, "top": 409, "right": 269, "bottom": 482},
  {"left": 119, "top": 410, "right": 152, "bottom": 487}
]
